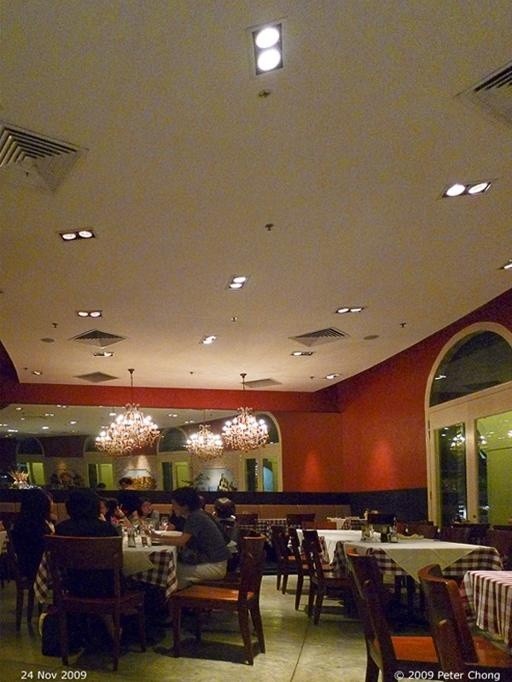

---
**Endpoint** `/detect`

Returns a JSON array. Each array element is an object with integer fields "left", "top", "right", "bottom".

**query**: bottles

[
  {"left": 127, "top": 526, "right": 148, "bottom": 548},
  {"left": 387, "top": 532, "right": 398, "bottom": 543},
  {"left": 391, "top": 516, "right": 397, "bottom": 530}
]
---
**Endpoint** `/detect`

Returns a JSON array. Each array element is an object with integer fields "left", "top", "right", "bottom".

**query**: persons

[
  {"left": 117, "top": 478, "right": 135, "bottom": 490},
  {"left": 96, "top": 484, "right": 106, "bottom": 491},
  {"left": 96, "top": 494, "right": 238, "bottom": 572},
  {"left": 146, "top": 487, "right": 230, "bottom": 632},
  {"left": 55, "top": 491, "right": 125, "bottom": 642},
  {"left": 11, "top": 489, "right": 59, "bottom": 579}
]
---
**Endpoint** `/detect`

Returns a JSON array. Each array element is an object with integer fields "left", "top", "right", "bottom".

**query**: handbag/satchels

[{"left": 42, "top": 615, "right": 86, "bottom": 655}]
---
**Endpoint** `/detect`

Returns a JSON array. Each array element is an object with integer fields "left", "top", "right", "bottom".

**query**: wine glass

[{"left": 139, "top": 516, "right": 168, "bottom": 536}]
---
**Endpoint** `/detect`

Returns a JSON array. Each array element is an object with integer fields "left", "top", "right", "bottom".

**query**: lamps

[
  {"left": 184, "top": 373, "right": 223, "bottom": 460},
  {"left": 98, "top": 368, "right": 160, "bottom": 454},
  {"left": 221, "top": 374, "right": 270, "bottom": 452}
]
